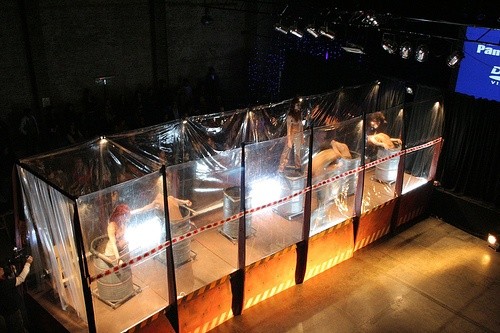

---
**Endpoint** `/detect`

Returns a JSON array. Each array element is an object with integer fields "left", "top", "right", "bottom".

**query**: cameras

[{"left": 4, "top": 245, "right": 29, "bottom": 276}]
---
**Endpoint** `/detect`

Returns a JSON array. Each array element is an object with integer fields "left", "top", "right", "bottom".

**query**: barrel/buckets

[
  {"left": 374, "top": 141, "right": 402, "bottom": 183},
  {"left": 222, "top": 184, "right": 253, "bottom": 239},
  {"left": 90, "top": 235, "right": 133, "bottom": 301},
  {"left": 277, "top": 166, "right": 305, "bottom": 214},
  {"left": 334, "top": 150, "right": 361, "bottom": 198},
  {"left": 154, "top": 204, "right": 192, "bottom": 266},
  {"left": 316, "top": 160, "right": 342, "bottom": 202}
]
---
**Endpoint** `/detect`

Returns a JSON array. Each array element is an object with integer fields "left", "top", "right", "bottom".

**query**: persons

[
  {"left": 0, "top": 256, "right": 35, "bottom": 333},
  {"left": 366, "top": 133, "right": 403, "bottom": 152},
  {"left": 305, "top": 138, "right": 353, "bottom": 177},
  {"left": 153, "top": 188, "right": 193, "bottom": 223},
  {"left": 104, "top": 202, "right": 158, "bottom": 268}
]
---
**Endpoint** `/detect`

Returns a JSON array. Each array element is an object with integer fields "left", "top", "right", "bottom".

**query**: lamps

[
  {"left": 288, "top": 25, "right": 304, "bottom": 38},
  {"left": 382, "top": 39, "right": 398, "bottom": 53},
  {"left": 273, "top": 23, "right": 289, "bottom": 35},
  {"left": 318, "top": 26, "right": 336, "bottom": 40},
  {"left": 400, "top": 42, "right": 412, "bottom": 60},
  {"left": 416, "top": 45, "right": 429, "bottom": 62},
  {"left": 446, "top": 51, "right": 464, "bottom": 67},
  {"left": 306, "top": 24, "right": 320, "bottom": 38}
]
